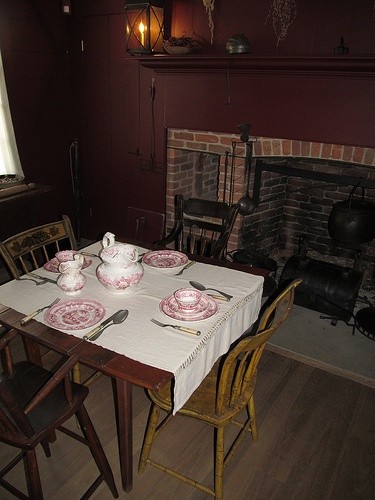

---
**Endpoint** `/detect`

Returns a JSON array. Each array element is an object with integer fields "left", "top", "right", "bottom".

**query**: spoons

[
  {"left": 189, "top": 281, "right": 233, "bottom": 298},
  {"left": 89, "top": 310, "right": 128, "bottom": 341}
]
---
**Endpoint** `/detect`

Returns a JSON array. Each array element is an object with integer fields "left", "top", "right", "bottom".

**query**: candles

[{"left": 139, "top": 21, "right": 144, "bottom": 49}]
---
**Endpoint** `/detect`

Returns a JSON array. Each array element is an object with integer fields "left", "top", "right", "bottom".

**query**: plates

[
  {"left": 160, "top": 292, "right": 218, "bottom": 322},
  {"left": 44, "top": 299, "right": 105, "bottom": 330},
  {"left": 142, "top": 250, "right": 188, "bottom": 269},
  {"left": 44, "top": 257, "right": 92, "bottom": 274}
]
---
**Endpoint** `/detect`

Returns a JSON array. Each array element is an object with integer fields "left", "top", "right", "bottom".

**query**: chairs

[
  {"left": 0, "top": 324, "right": 120, "bottom": 500},
  {"left": 138, "top": 279, "right": 300, "bottom": 500},
  {"left": 0, "top": 215, "right": 102, "bottom": 385},
  {"left": 160, "top": 193, "right": 236, "bottom": 259}
]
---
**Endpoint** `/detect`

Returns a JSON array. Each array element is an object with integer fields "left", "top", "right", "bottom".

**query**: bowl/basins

[
  {"left": 174, "top": 288, "right": 202, "bottom": 311},
  {"left": 55, "top": 250, "right": 77, "bottom": 263},
  {"left": 164, "top": 46, "right": 197, "bottom": 55}
]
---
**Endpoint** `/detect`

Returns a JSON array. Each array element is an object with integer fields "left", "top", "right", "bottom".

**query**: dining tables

[{"left": 0, "top": 240, "right": 269, "bottom": 494}]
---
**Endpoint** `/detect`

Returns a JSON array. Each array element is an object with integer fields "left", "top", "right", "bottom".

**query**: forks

[
  {"left": 151, "top": 318, "right": 201, "bottom": 336},
  {"left": 21, "top": 298, "right": 60, "bottom": 325},
  {"left": 16, "top": 277, "right": 49, "bottom": 286},
  {"left": 176, "top": 260, "right": 196, "bottom": 276}
]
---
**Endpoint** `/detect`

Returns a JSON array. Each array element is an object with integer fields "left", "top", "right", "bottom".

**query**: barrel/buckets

[{"left": 328, "top": 182, "right": 375, "bottom": 244}]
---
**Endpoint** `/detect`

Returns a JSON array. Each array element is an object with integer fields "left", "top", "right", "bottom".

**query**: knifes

[
  {"left": 27, "top": 272, "right": 57, "bottom": 284},
  {"left": 83, "top": 310, "right": 123, "bottom": 340}
]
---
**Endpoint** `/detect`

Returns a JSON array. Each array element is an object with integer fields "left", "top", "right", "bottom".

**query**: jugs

[
  {"left": 95, "top": 232, "right": 144, "bottom": 293},
  {"left": 56, "top": 255, "right": 87, "bottom": 293}
]
